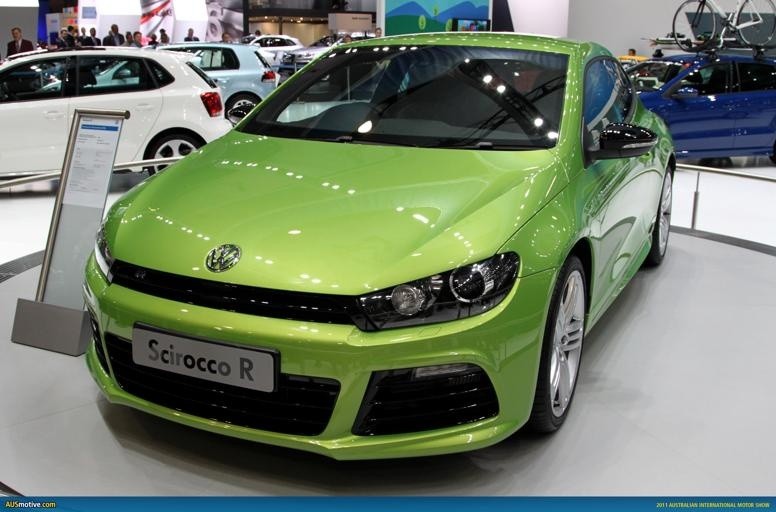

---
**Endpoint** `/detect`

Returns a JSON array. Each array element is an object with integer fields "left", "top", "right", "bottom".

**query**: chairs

[
  {"left": 685, "top": 71, "right": 703, "bottom": 93},
  {"left": 705, "top": 70, "right": 727, "bottom": 93}
]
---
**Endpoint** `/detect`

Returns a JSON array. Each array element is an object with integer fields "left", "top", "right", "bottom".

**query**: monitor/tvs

[{"left": 452, "top": 18, "right": 491, "bottom": 31}]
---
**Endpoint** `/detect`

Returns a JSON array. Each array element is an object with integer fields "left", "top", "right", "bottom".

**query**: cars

[
  {"left": 145, "top": 32, "right": 376, "bottom": 120},
  {"left": 0, "top": 45, "right": 233, "bottom": 183}
]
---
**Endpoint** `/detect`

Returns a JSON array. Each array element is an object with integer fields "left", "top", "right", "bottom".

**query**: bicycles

[{"left": 671, "top": 0, "right": 776, "bottom": 51}]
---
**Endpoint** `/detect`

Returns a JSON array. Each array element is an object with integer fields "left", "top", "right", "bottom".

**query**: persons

[
  {"left": 6, "top": 27, "right": 34, "bottom": 55},
  {"left": 220, "top": 32, "right": 232, "bottom": 42},
  {"left": 36, "top": 24, "right": 169, "bottom": 48},
  {"left": 255, "top": 30, "right": 261, "bottom": 37},
  {"left": 375, "top": 27, "right": 382, "bottom": 39},
  {"left": 628, "top": 49, "right": 636, "bottom": 56},
  {"left": 344, "top": 35, "right": 353, "bottom": 43},
  {"left": 184, "top": 28, "right": 199, "bottom": 41}
]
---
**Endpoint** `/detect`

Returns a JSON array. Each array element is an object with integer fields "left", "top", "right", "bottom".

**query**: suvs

[{"left": 622, "top": 53, "right": 775, "bottom": 164}]
